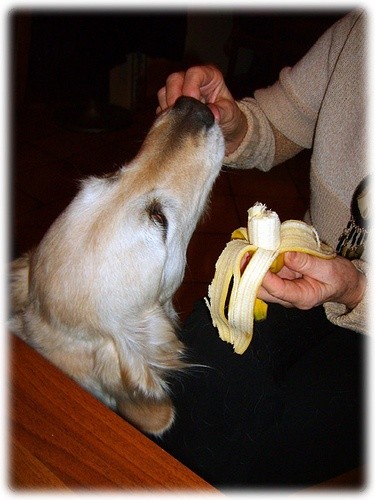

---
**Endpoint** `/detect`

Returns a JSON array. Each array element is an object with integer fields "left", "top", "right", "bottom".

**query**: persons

[{"left": 109, "top": 6, "right": 368, "bottom": 488}]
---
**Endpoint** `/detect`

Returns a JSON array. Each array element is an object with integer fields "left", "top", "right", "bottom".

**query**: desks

[{"left": 10, "top": 328, "right": 224, "bottom": 495}]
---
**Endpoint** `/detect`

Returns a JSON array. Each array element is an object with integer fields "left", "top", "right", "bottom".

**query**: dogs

[{"left": 7, "top": 95, "right": 227, "bottom": 441}]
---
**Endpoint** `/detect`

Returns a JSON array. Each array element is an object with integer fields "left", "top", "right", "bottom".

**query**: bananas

[{"left": 207, "top": 202, "right": 336, "bottom": 355}]
